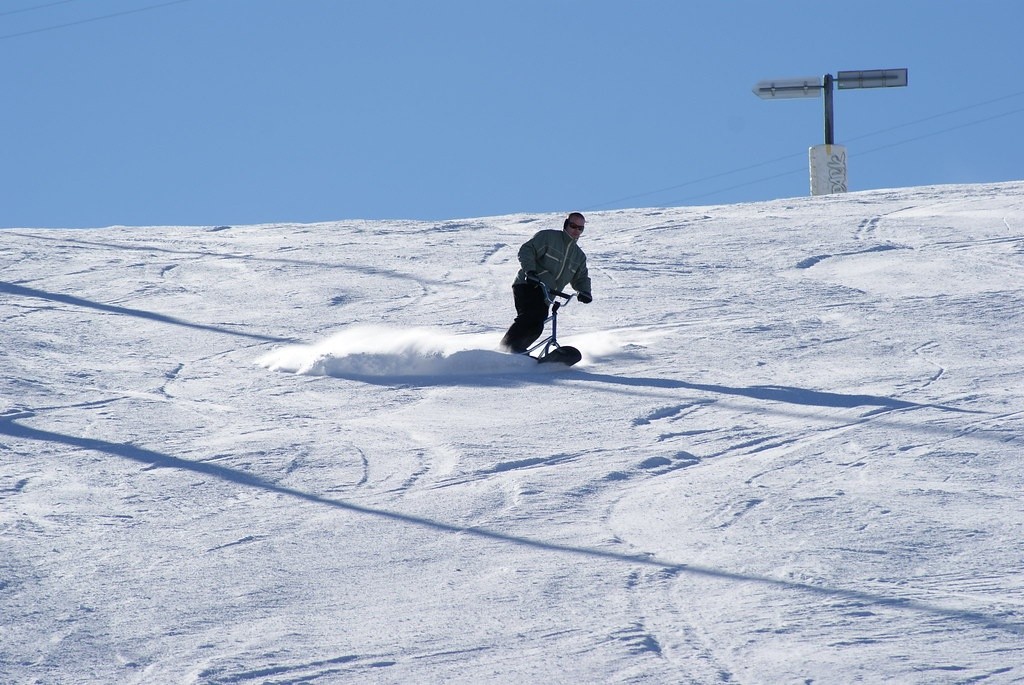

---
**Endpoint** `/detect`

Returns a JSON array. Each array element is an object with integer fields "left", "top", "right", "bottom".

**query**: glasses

[{"left": 568, "top": 221, "right": 584, "bottom": 231}]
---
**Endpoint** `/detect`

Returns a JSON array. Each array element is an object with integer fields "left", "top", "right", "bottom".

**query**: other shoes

[{"left": 496, "top": 344, "right": 530, "bottom": 356}]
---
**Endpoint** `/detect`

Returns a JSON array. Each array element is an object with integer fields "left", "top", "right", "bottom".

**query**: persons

[{"left": 500, "top": 213, "right": 592, "bottom": 356}]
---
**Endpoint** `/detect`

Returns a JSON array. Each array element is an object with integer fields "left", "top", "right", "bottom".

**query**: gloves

[
  {"left": 578, "top": 291, "right": 592, "bottom": 304},
  {"left": 525, "top": 271, "right": 540, "bottom": 287}
]
